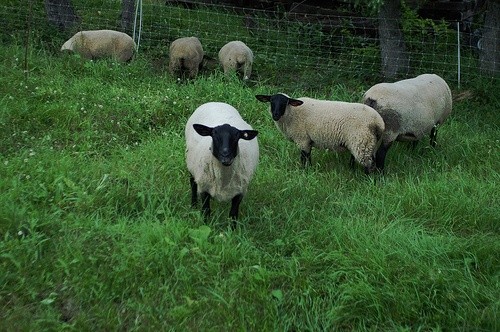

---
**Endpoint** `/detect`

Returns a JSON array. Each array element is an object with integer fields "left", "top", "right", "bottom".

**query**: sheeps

[
  {"left": 218, "top": 41, "right": 254, "bottom": 84},
  {"left": 361, "top": 73, "right": 453, "bottom": 173},
  {"left": 255, "top": 92, "right": 386, "bottom": 179},
  {"left": 184, "top": 102, "right": 260, "bottom": 231},
  {"left": 61, "top": 30, "right": 137, "bottom": 64},
  {"left": 169, "top": 36, "right": 212, "bottom": 80}
]
